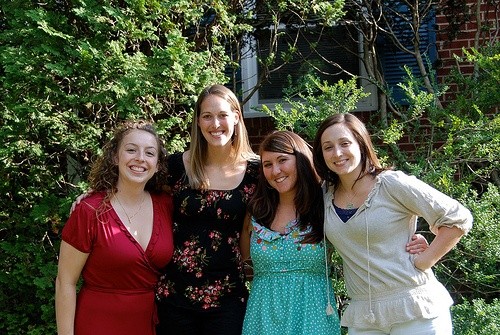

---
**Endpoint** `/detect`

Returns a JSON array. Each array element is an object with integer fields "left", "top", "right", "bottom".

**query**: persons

[
  {"left": 55, "top": 120, "right": 174, "bottom": 335},
  {"left": 69, "top": 85, "right": 260, "bottom": 335},
  {"left": 313, "top": 112, "right": 473, "bottom": 335},
  {"left": 240, "top": 130, "right": 429, "bottom": 334}
]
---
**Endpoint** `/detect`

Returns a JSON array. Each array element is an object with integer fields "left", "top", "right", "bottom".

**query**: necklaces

[
  {"left": 340, "top": 171, "right": 367, "bottom": 209},
  {"left": 114, "top": 192, "right": 146, "bottom": 223}
]
